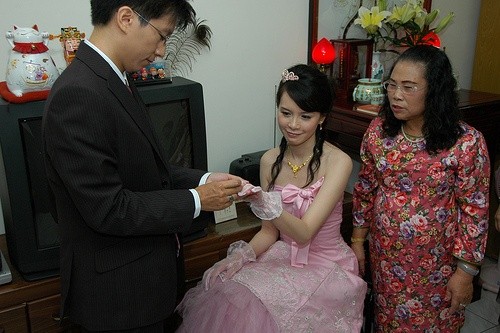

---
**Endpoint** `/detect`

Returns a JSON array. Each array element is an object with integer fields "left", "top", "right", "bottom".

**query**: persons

[
  {"left": 173, "top": 63, "right": 368, "bottom": 333},
  {"left": 494, "top": 167, "right": 500, "bottom": 232},
  {"left": 41, "top": 1, "right": 242, "bottom": 333},
  {"left": 352, "top": 44, "right": 486, "bottom": 333}
]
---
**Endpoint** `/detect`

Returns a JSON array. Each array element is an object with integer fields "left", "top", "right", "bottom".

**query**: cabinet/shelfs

[{"left": 0, "top": 191, "right": 354, "bottom": 333}]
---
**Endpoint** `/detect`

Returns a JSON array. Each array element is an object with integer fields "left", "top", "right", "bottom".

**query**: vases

[{"left": 362, "top": 50, "right": 385, "bottom": 84}]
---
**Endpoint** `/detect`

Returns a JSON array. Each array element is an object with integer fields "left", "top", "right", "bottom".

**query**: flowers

[{"left": 353, "top": 0, "right": 455, "bottom": 57}]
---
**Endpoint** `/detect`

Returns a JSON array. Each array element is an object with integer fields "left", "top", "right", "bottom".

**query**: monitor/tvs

[{"left": 0, "top": 76, "right": 216, "bottom": 282}]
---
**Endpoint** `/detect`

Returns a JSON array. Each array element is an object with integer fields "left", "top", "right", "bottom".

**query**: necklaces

[
  {"left": 457, "top": 260, "right": 479, "bottom": 275},
  {"left": 286, "top": 147, "right": 314, "bottom": 176}
]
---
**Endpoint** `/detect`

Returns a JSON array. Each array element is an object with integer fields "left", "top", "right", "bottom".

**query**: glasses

[
  {"left": 131, "top": 7, "right": 169, "bottom": 45},
  {"left": 383, "top": 80, "right": 430, "bottom": 95}
]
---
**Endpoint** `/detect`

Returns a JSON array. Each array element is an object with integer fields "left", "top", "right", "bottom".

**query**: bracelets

[{"left": 350, "top": 237, "right": 366, "bottom": 242}]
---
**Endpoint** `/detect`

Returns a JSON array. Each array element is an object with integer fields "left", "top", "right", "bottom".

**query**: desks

[{"left": 325, "top": 88, "right": 500, "bottom": 263}]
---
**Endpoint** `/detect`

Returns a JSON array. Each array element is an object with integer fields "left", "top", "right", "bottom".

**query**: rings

[
  {"left": 228, "top": 195, "right": 234, "bottom": 203},
  {"left": 460, "top": 304, "right": 467, "bottom": 307}
]
